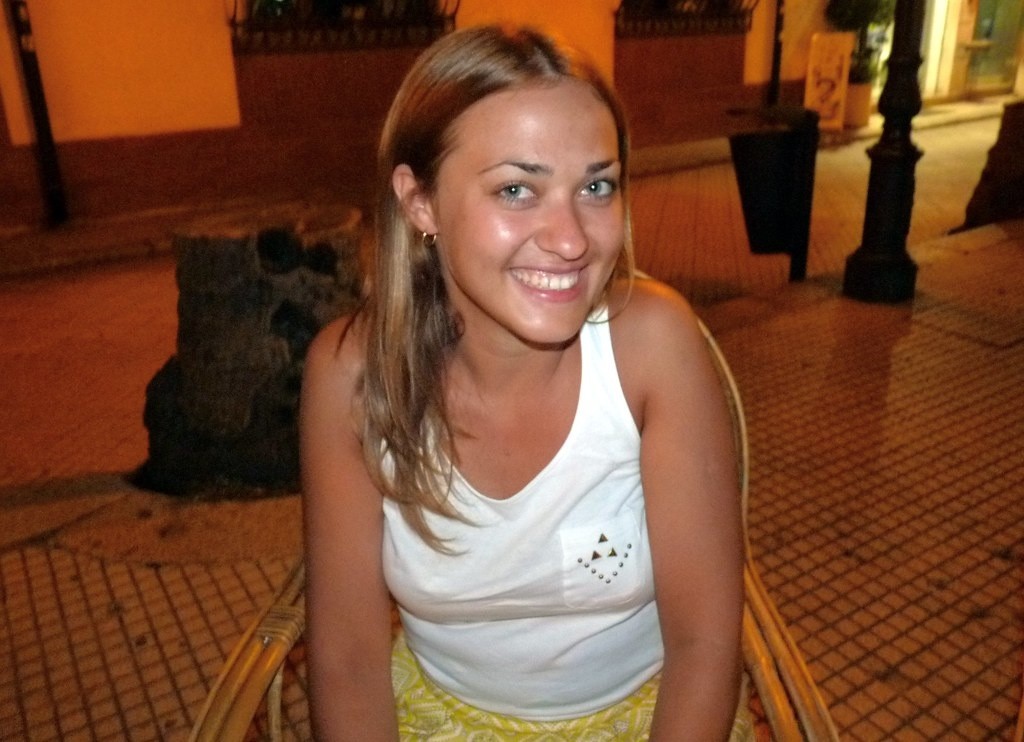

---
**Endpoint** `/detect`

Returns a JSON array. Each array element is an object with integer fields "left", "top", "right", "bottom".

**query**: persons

[{"left": 297, "top": 25, "right": 746, "bottom": 742}]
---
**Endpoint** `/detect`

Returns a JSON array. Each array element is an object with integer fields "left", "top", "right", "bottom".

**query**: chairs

[{"left": 187, "top": 265, "right": 839, "bottom": 742}]
